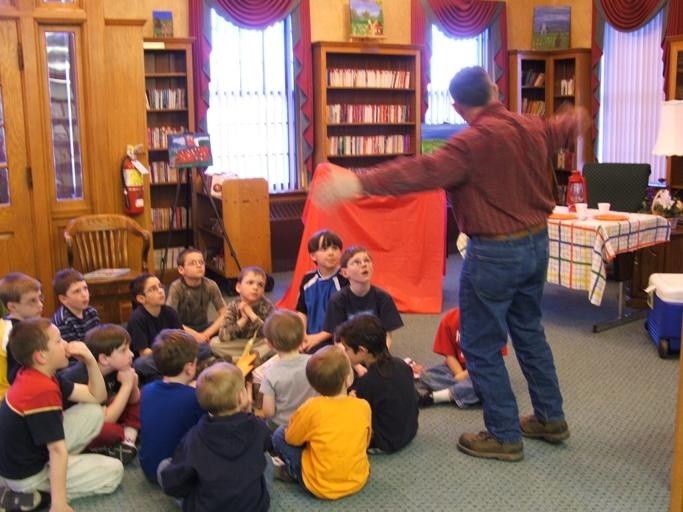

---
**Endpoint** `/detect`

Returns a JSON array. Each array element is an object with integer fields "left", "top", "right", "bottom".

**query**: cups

[
  {"left": 575, "top": 203, "right": 587, "bottom": 221},
  {"left": 598, "top": 202, "right": 610, "bottom": 215}
]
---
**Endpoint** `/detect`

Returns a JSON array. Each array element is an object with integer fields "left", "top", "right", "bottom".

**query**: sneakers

[
  {"left": 1, "top": 488, "right": 50, "bottom": 511},
  {"left": 90, "top": 442, "right": 136, "bottom": 466}
]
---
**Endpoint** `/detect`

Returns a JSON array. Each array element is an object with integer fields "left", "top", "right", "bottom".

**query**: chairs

[
  {"left": 582, "top": 164, "right": 651, "bottom": 284},
  {"left": 64, "top": 214, "right": 154, "bottom": 323}
]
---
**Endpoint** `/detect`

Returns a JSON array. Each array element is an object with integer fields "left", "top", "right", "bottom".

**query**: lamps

[{"left": 652, "top": 99, "right": 682, "bottom": 155}]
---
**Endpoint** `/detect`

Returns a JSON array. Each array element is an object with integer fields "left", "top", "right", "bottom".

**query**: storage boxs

[{"left": 644, "top": 273, "right": 683, "bottom": 361}]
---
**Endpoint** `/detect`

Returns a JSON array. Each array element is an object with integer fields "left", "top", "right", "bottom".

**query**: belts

[{"left": 477, "top": 222, "right": 548, "bottom": 241}]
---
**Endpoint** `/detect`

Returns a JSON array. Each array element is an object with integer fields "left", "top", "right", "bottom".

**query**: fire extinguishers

[{"left": 120, "top": 144, "right": 148, "bottom": 214}]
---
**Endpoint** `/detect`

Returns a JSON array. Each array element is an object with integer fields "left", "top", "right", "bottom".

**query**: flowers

[{"left": 654, "top": 189, "right": 678, "bottom": 217}]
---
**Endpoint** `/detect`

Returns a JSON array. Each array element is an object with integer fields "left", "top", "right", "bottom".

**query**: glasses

[
  {"left": 12, "top": 294, "right": 44, "bottom": 307},
  {"left": 345, "top": 258, "right": 373, "bottom": 266},
  {"left": 141, "top": 284, "right": 162, "bottom": 295},
  {"left": 183, "top": 260, "right": 205, "bottom": 267}
]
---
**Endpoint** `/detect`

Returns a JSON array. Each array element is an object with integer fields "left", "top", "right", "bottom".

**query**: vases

[{"left": 666, "top": 217, "right": 679, "bottom": 229}]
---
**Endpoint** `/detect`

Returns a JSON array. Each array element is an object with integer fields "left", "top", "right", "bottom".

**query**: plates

[
  {"left": 595, "top": 214, "right": 629, "bottom": 221},
  {"left": 548, "top": 214, "right": 578, "bottom": 219}
]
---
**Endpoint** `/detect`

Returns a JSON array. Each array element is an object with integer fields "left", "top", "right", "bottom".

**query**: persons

[
  {"left": 272, "top": 345, "right": 373, "bottom": 500},
  {"left": 259, "top": 311, "right": 318, "bottom": 434},
  {"left": 0, "top": 272, "right": 46, "bottom": 405},
  {"left": 322, "top": 245, "right": 404, "bottom": 378},
  {"left": 2, "top": 319, "right": 127, "bottom": 512},
  {"left": 55, "top": 323, "right": 141, "bottom": 462},
  {"left": 51, "top": 269, "right": 100, "bottom": 347},
  {"left": 155, "top": 362, "right": 274, "bottom": 511},
  {"left": 418, "top": 306, "right": 509, "bottom": 408},
  {"left": 166, "top": 249, "right": 227, "bottom": 345},
  {"left": 138, "top": 328, "right": 206, "bottom": 486},
  {"left": 209, "top": 268, "right": 276, "bottom": 367},
  {"left": 128, "top": 275, "right": 212, "bottom": 381},
  {"left": 296, "top": 230, "right": 350, "bottom": 356},
  {"left": 312, "top": 64, "right": 591, "bottom": 462},
  {"left": 337, "top": 314, "right": 420, "bottom": 453}
]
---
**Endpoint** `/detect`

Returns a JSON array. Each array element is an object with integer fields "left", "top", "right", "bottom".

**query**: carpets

[{"left": 68, "top": 282, "right": 682, "bottom": 512}]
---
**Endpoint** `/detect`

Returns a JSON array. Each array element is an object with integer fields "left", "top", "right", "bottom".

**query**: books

[
  {"left": 153, "top": 245, "right": 184, "bottom": 267},
  {"left": 331, "top": 135, "right": 407, "bottom": 155},
  {"left": 328, "top": 68, "right": 412, "bottom": 91},
  {"left": 555, "top": 150, "right": 576, "bottom": 171},
  {"left": 148, "top": 162, "right": 188, "bottom": 181},
  {"left": 559, "top": 78, "right": 574, "bottom": 96},
  {"left": 150, "top": 207, "right": 188, "bottom": 231},
  {"left": 523, "top": 70, "right": 549, "bottom": 88},
  {"left": 145, "top": 53, "right": 185, "bottom": 74},
  {"left": 141, "top": 87, "right": 188, "bottom": 111},
  {"left": 148, "top": 124, "right": 186, "bottom": 151},
  {"left": 332, "top": 103, "right": 411, "bottom": 125},
  {"left": 522, "top": 96, "right": 545, "bottom": 119},
  {"left": 555, "top": 184, "right": 568, "bottom": 206}
]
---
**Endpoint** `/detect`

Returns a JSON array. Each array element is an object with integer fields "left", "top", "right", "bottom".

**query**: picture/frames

[
  {"left": 152, "top": 10, "right": 173, "bottom": 38},
  {"left": 531, "top": 6, "right": 571, "bottom": 50},
  {"left": 349, "top": 0, "right": 384, "bottom": 38},
  {"left": 167, "top": 133, "right": 212, "bottom": 168}
]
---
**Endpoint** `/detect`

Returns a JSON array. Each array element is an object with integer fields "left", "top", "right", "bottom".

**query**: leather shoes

[
  {"left": 416, "top": 390, "right": 433, "bottom": 407},
  {"left": 519, "top": 415, "right": 570, "bottom": 441},
  {"left": 458, "top": 430, "right": 524, "bottom": 461}
]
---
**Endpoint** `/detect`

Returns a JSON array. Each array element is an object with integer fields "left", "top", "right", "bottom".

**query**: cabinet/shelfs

[
  {"left": 312, "top": 41, "right": 425, "bottom": 169},
  {"left": 508, "top": 49, "right": 591, "bottom": 204},
  {"left": 142, "top": 37, "right": 197, "bottom": 271},
  {"left": 197, "top": 178, "right": 271, "bottom": 278},
  {"left": 665, "top": 41, "right": 682, "bottom": 197}
]
